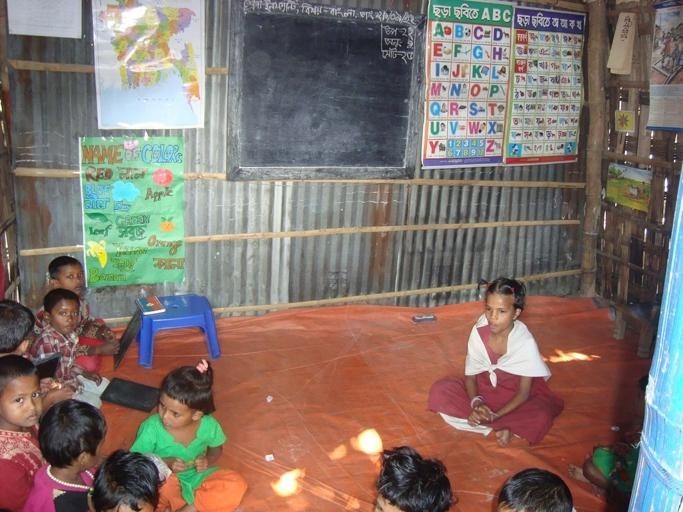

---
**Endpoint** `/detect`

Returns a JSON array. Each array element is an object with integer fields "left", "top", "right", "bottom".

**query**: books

[{"left": 135, "top": 295, "right": 165, "bottom": 316}]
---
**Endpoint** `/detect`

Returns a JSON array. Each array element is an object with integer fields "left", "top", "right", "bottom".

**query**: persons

[
  {"left": 373, "top": 444, "right": 453, "bottom": 512},
  {"left": 427, "top": 278, "right": 565, "bottom": 448},
  {"left": 127, "top": 357, "right": 250, "bottom": 511},
  {"left": 1, "top": 253, "right": 163, "bottom": 511},
  {"left": 581, "top": 374, "right": 650, "bottom": 504},
  {"left": 494, "top": 467, "right": 574, "bottom": 511}
]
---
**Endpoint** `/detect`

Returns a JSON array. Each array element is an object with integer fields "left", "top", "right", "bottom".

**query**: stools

[
  {"left": 139, "top": 294, "right": 222, "bottom": 368},
  {"left": 610, "top": 302, "right": 657, "bottom": 358}
]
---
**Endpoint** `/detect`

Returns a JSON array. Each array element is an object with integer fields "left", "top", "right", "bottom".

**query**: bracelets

[
  {"left": 490, "top": 413, "right": 494, "bottom": 423},
  {"left": 470, "top": 396, "right": 485, "bottom": 409}
]
